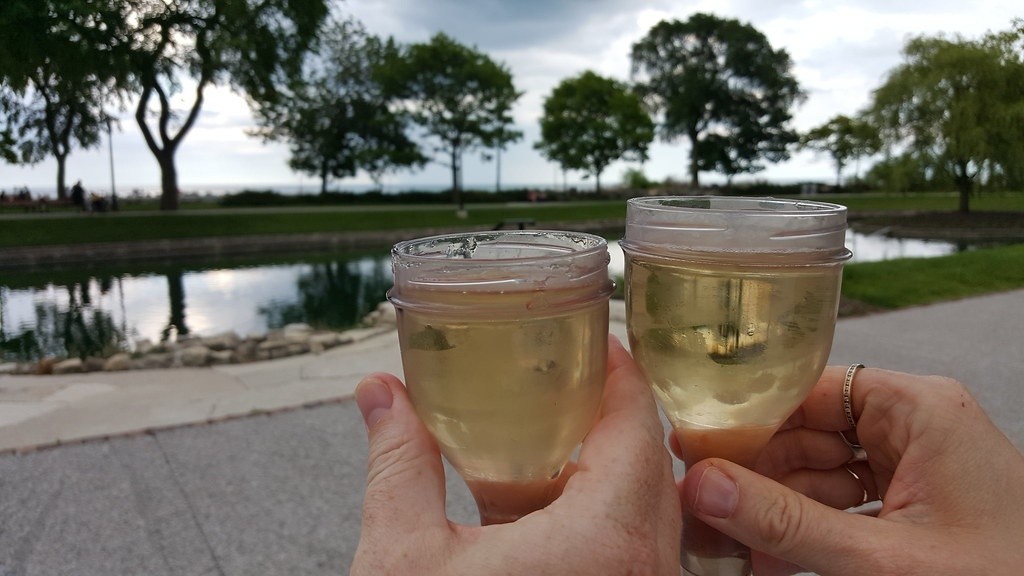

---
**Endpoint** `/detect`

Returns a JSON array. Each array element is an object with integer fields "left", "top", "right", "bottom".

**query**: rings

[
  {"left": 838, "top": 431, "right": 867, "bottom": 465},
  {"left": 840, "top": 364, "right": 866, "bottom": 428},
  {"left": 844, "top": 466, "right": 867, "bottom": 507}
]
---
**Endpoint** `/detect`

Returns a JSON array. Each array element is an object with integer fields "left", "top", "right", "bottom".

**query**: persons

[
  {"left": 669, "top": 361, "right": 1022, "bottom": 576},
  {"left": 347, "top": 331, "right": 682, "bottom": 575}
]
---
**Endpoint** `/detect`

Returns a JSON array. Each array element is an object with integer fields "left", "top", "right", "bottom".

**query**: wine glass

[
  {"left": 386, "top": 229, "right": 619, "bottom": 527},
  {"left": 622, "top": 196, "right": 854, "bottom": 576}
]
